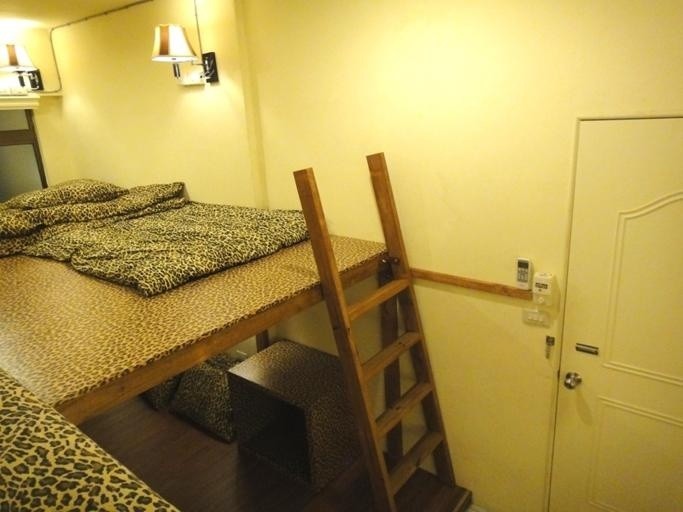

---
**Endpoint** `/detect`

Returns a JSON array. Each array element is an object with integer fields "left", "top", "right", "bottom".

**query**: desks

[{"left": 227, "top": 337, "right": 362, "bottom": 494}]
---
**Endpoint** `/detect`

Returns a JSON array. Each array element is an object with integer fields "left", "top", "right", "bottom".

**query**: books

[{"left": 0, "top": 87, "right": 40, "bottom": 110}]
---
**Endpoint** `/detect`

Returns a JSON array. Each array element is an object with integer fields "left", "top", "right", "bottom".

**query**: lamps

[
  {"left": 1, "top": 44, "right": 44, "bottom": 92},
  {"left": 151, "top": 25, "right": 219, "bottom": 86}
]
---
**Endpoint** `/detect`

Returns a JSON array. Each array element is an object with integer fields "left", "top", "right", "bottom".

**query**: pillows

[
  {"left": 5, "top": 178, "right": 130, "bottom": 212},
  {"left": 142, "top": 352, "right": 244, "bottom": 444}
]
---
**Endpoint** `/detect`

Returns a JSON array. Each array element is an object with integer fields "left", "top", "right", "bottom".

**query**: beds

[{"left": 0, "top": 188, "right": 390, "bottom": 406}]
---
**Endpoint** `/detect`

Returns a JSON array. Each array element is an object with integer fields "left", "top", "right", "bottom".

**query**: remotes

[{"left": 516, "top": 258, "right": 531, "bottom": 290}]
